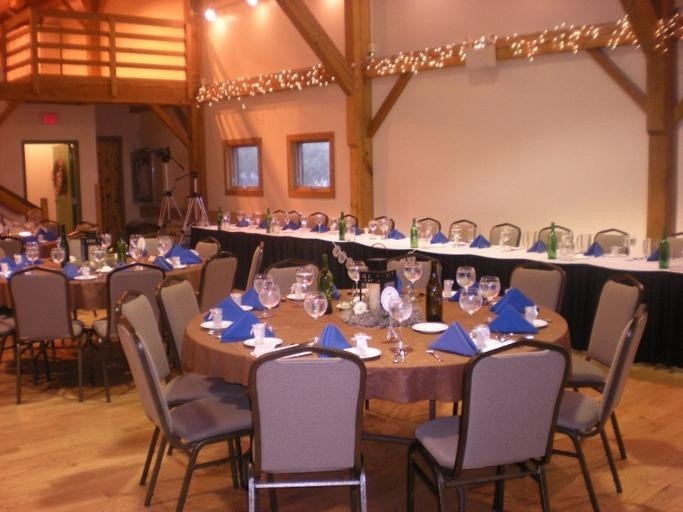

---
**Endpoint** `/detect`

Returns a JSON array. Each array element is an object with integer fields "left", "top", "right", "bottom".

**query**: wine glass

[
  {"left": 625, "top": 235, "right": 637, "bottom": 261},
  {"left": 254, "top": 209, "right": 263, "bottom": 230},
  {"left": 155, "top": 236, "right": 172, "bottom": 257},
  {"left": 459, "top": 288, "right": 482, "bottom": 332},
  {"left": 128, "top": 234, "right": 145, "bottom": 271},
  {"left": 100, "top": 233, "right": 112, "bottom": 249},
  {"left": 315, "top": 216, "right": 323, "bottom": 234},
  {"left": 25, "top": 241, "right": 40, "bottom": 273},
  {"left": 403, "top": 263, "right": 423, "bottom": 302},
  {"left": 296, "top": 267, "right": 315, "bottom": 298},
  {"left": 235, "top": 208, "right": 245, "bottom": 228},
  {"left": 259, "top": 284, "right": 281, "bottom": 334},
  {"left": 348, "top": 261, "right": 365, "bottom": 295},
  {"left": 300, "top": 216, "right": 309, "bottom": 233},
  {"left": 456, "top": 267, "right": 476, "bottom": 293},
  {"left": 50, "top": 248, "right": 66, "bottom": 269},
  {"left": 254, "top": 274, "right": 275, "bottom": 317},
  {"left": 479, "top": 276, "right": 501, "bottom": 322},
  {"left": 304, "top": 291, "right": 328, "bottom": 346},
  {"left": 388, "top": 295, "right": 413, "bottom": 363},
  {"left": 93, "top": 251, "right": 107, "bottom": 278},
  {"left": 368, "top": 220, "right": 378, "bottom": 240},
  {"left": 379, "top": 219, "right": 389, "bottom": 242}
]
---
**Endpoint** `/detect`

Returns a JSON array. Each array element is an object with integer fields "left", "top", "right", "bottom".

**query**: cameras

[{"left": 156, "top": 147, "right": 171, "bottom": 163}]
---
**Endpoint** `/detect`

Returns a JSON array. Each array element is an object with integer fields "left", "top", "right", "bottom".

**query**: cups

[
  {"left": 354, "top": 333, "right": 368, "bottom": 355},
  {"left": 444, "top": 279, "right": 452, "bottom": 296},
  {"left": 253, "top": 324, "right": 265, "bottom": 345},
  {"left": 211, "top": 308, "right": 223, "bottom": 326},
  {"left": 525, "top": 307, "right": 535, "bottom": 323}
]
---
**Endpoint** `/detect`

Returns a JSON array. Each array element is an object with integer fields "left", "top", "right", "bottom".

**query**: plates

[
  {"left": 243, "top": 337, "right": 283, "bottom": 346},
  {"left": 200, "top": 321, "right": 233, "bottom": 329},
  {"left": 343, "top": 347, "right": 382, "bottom": 359},
  {"left": 534, "top": 319, "right": 548, "bottom": 328},
  {"left": 238, "top": 305, "right": 253, "bottom": 311},
  {"left": 470, "top": 327, "right": 520, "bottom": 352},
  {"left": 442, "top": 291, "right": 457, "bottom": 297},
  {"left": 286, "top": 294, "right": 306, "bottom": 300},
  {"left": 412, "top": 323, "right": 448, "bottom": 333},
  {"left": 73, "top": 275, "right": 98, "bottom": 280}
]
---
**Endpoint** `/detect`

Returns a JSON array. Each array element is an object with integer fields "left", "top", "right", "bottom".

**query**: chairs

[
  {"left": 417, "top": 218, "right": 441, "bottom": 237},
  {"left": 307, "top": 212, "right": 327, "bottom": 229},
  {"left": 387, "top": 252, "right": 443, "bottom": 289},
  {"left": 538, "top": 225, "right": 572, "bottom": 247},
  {"left": 264, "top": 259, "right": 320, "bottom": 296},
  {"left": 35, "top": 219, "right": 59, "bottom": 239},
  {"left": 659, "top": 232, "right": 683, "bottom": 257},
  {"left": 506, "top": 262, "right": 566, "bottom": 314},
  {"left": 140, "top": 232, "right": 159, "bottom": 255},
  {"left": 245, "top": 241, "right": 264, "bottom": 293},
  {"left": 448, "top": 219, "right": 476, "bottom": 243},
  {"left": 115, "top": 314, "right": 252, "bottom": 511},
  {"left": 374, "top": 216, "right": 395, "bottom": 234},
  {"left": 75, "top": 220, "right": 99, "bottom": 233},
  {"left": 569, "top": 275, "right": 644, "bottom": 457},
  {"left": 195, "top": 235, "right": 221, "bottom": 261},
  {"left": 489, "top": 223, "right": 521, "bottom": 247},
  {"left": 67, "top": 229, "right": 96, "bottom": 262},
  {"left": 405, "top": 340, "right": 571, "bottom": 512},
  {"left": 200, "top": 250, "right": 240, "bottom": 315},
  {"left": 88, "top": 262, "right": 165, "bottom": 403},
  {"left": 115, "top": 288, "right": 248, "bottom": 486},
  {"left": 593, "top": 228, "right": 629, "bottom": 255},
  {"left": 288, "top": 210, "right": 301, "bottom": 225},
  {"left": 272, "top": 210, "right": 286, "bottom": 223},
  {"left": 9, "top": 266, "right": 86, "bottom": 405},
  {"left": 156, "top": 276, "right": 201, "bottom": 374},
  {"left": 0, "top": 235, "right": 22, "bottom": 261},
  {"left": 241, "top": 345, "right": 368, "bottom": 512},
  {"left": 338, "top": 214, "right": 358, "bottom": 229},
  {"left": 0, "top": 314, "right": 38, "bottom": 384},
  {"left": 556, "top": 301, "right": 647, "bottom": 511}
]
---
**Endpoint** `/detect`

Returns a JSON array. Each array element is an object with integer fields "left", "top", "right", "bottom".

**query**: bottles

[
  {"left": 547, "top": 222, "right": 558, "bottom": 259},
  {"left": 115, "top": 230, "right": 129, "bottom": 264},
  {"left": 426, "top": 260, "right": 443, "bottom": 322},
  {"left": 410, "top": 218, "right": 418, "bottom": 248},
  {"left": 216, "top": 206, "right": 223, "bottom": 230},
  {"left": 264, "top": 208, "right": 272, "bottom": 233},
  {"left": 60, "top": 224, "right": 69, "bottom": 261},
  {"left": 659, "top": 224, "right": 670, "bottom": 269},
  {"left": 318, "top": 254, "right": 333, "bottom": 314},
  {"left": 338, "top": 212, "right": 346, "bottom": 240}
]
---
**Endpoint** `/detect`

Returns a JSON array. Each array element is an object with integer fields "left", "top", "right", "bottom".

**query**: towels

[
  {"left": 10, "top": 263, "right": 30, "bottom": 277},
  {"left": 236, "top": 220, "right": 249, "bottom": 227},
  {"left": 18, "top": 252, "right": 42, "bottom": 265},
  {"left": 43, "top": 227, "right": 57, "bottom": 239},
  {"left": 488, "top": 305, "right": 538, "bottom": 333},
  {"left": 220, "top": 311, "right": 275, "bottom": 341},
  {"left": 205, "top": 295, "right": 244, "bottom": 321},
  {"left": 241, "top": 286, "right": 265, "bottom": 311},
  {"left": 151, "top": 255, "right": 171, "bottom": 271},
  {"left": 648, "top": 247, "right": 660, "bottom": 262},
  {"left": 448, "top": 280, "right": 486, "bottom": 302},
  {"left": 387, "top": 230, "right": 406, "bottom": 238},
  {"left": 431, "top": 231, "right": 450, "bottom": 244},
  {"left": 330, "top": 283, "right": 341, "bottom": 298},
  {"left": 257, "top": 219, "right": 267, "bottom": 228},
  {"left": 60, "top": 262, "right": 79, "bottom": 277},
  {"left": 0, "top": 255, "right": 17, "bottom": 271},
  {"left": 281, "top": 220, "right": 300, "bottom": 230},
  {"left": 319, "top": 323, "right": 351, "bottom": 357},
  {"left": 176, "top": 248, "right": 203, "bottom": 264},
  {"left": 469, "top": 235, "right": 492, "bottom": 248},
  {"left": 491, "top": 287, "right": 539, "bottom": 312},
  {"left": 166, "top": 244, "right": 187, "bottom": 257},
  {"left": 309, "top": 224, "right": 329, "bottom": 233},
  {"left": 348, "top": 224, "right": 361, "bottom": 236},
  {"left": 23, "top": 235, "right": 38, "bottom": 244},
  {"left": 428, "top": 322, "right": 478, "bottom": 357},
  {"left": 526, "top": 240, "right": 549, "bottom": 252},
  {"left": 396, "top": 274, "right": 403, "bottom": 293},
  {"left": 583, "top": 242, "right": 604, "bottom": 256}
]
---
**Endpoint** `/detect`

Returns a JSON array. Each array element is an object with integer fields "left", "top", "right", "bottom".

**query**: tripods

[
  {"left": 177, "top": 171, "right": 211, "bottom": 247},
  {"left": 156, "top": 163, "right": 184, "bottom": 235}
]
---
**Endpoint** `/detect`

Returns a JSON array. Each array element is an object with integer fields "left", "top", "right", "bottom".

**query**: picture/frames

[{"left": 130, "top": 149, "right": 154, "bottom": 203}]
